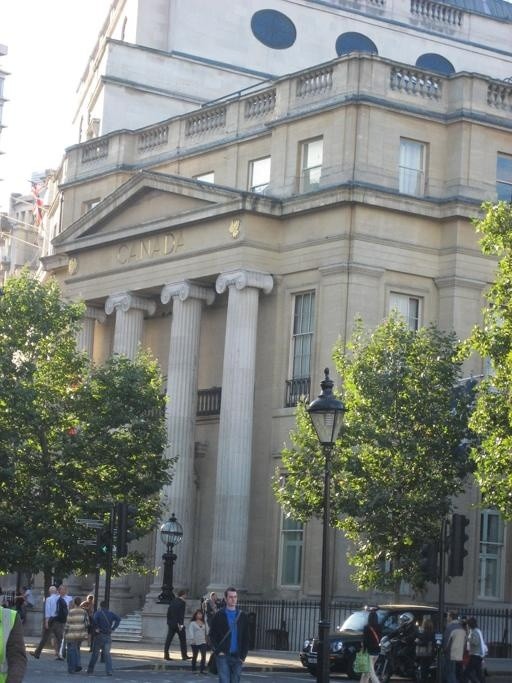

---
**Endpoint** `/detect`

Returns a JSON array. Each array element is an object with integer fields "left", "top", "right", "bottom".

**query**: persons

[
  {"left": 0, "top": 583, "right": 120, "bottom": 683},
  {"left": 359, "top": 609, "right": 488, "bottom": 683},
  {"left": 164, "top": 586, "right": 250, "bottom": 683}
]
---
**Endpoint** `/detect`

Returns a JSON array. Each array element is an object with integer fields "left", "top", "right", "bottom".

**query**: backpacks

[{"left": 56, "top": 597, "right": 68, "bottom": 623}]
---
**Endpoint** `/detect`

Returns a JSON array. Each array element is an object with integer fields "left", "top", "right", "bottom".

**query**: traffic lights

[
  {"left": 119, "top": 502, "right": 136, "bottom": 555},
  {"left": 450, "top": 514, "right": 470, "bottom": 576}
]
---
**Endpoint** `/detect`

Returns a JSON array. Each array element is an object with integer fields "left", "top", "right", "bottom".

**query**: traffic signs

[
  {"left": 87, "top": 522, "right": 103, "bottom": 530},
  {"left": 77, "top": 539, "right": 98, "bottom": 546},
  {"left": 73, "top": 518, "right": 103, "bottom": 525}
]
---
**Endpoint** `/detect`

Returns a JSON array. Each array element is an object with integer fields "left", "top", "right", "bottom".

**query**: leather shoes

[{"left": 30, "top": 652, "right": 39, "bottom": 659}]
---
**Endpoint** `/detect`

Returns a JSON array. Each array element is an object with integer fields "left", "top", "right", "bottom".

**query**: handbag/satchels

[
  {"left": 208, "top": 653, "right": 218, "bottom": 675},
  {"left": 353, "top": 652, "right": 370, "bottom": 673},
  {"left": 416, "top": 641, "right": 433, "bottom": 658}
]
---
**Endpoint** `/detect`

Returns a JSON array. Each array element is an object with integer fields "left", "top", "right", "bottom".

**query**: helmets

[{"left": 400, "top": 612, "right": 415, "bottom": 626}]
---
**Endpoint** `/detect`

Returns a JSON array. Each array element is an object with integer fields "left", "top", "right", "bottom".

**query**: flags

[{"left": 30, "top": 185, "right": 43, "bottom": 225}]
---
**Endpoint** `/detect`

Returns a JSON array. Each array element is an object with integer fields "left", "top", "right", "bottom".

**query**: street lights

[
  {"left": 156, "top": 512, "right": 185, "bottom": 604},
  {"left": 309, "top": 366, "right": 347, "bottom": 680}
]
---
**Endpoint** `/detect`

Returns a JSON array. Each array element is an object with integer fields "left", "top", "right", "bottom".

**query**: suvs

[{"left": 301, "top": 602, "right": 449, "bottom": 682}]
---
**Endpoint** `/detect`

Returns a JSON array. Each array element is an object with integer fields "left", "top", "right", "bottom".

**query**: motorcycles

[{"left": 373, "top": 630, "right": 434, "bottom": 682}]
